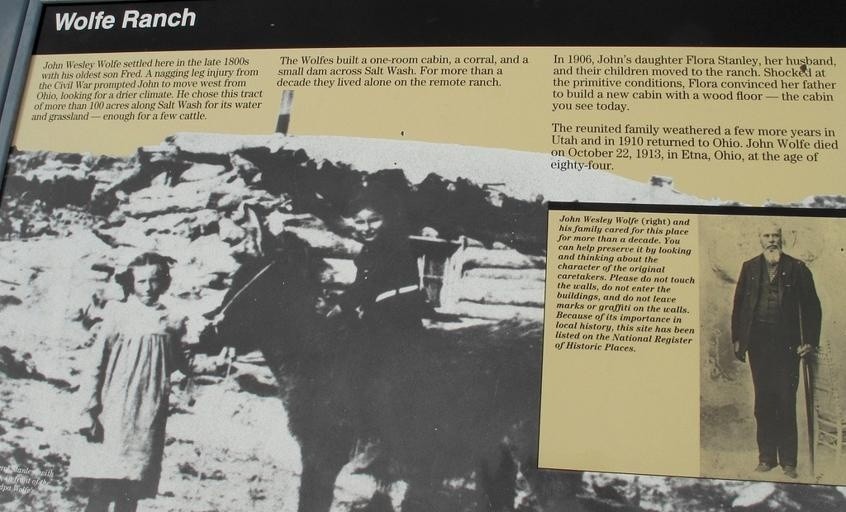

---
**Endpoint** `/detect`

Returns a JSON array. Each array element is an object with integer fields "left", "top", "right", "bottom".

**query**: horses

[{"left": 200, "top": 203, "right": 583, "bottom": 512}]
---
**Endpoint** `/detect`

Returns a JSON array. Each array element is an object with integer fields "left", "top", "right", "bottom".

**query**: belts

[{"left": 375, "top": 285, "right": 419, "bottom": 303}]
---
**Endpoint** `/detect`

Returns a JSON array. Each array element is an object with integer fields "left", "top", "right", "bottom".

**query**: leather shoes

[
  {"left": 755, "top": 463, "right": 778, "bottom": 472},
  {"left": 781, "top": 465, "right": 796, "bottom": 479}
]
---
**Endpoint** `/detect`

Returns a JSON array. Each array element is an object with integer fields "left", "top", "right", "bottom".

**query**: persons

[
  {"left": 732, "top": 221, "right": 821, "bottom": 479},
  {"left": 68, "top": 252, "right": 183, "bottom": 512},
  {"left": 320, "top": 198, "right": 428, "bottom": 472}
]
